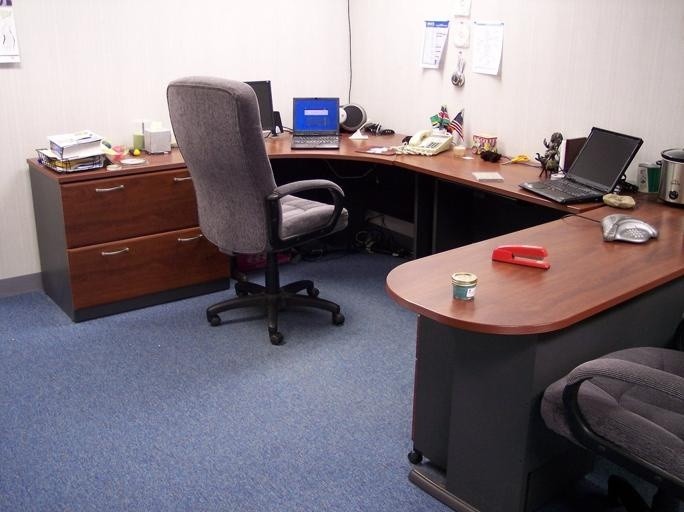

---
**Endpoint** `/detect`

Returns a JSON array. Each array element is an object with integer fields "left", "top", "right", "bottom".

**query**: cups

[{"left": 452, "top": 146, "right": 465, "bottom": 161}]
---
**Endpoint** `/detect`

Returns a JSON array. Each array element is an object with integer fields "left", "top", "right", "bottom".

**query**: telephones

[
  {"left": 601, "top": 213, "right": 658, "bottom": 244},
  {"left": 405, "top": 128, "right": 452, "bottom": 155}
]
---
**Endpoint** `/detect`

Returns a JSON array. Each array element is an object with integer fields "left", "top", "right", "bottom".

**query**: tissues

[{"left": 144, "top": 120, "right": 172, "bottom": 153}]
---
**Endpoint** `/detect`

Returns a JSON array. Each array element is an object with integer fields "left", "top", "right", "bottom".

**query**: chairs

[
  {"left": 539, "top": 344, "right": 683, "bottom": 511},
  {"left": 164, "top": 77, "right": 356, "bottom": 347}
]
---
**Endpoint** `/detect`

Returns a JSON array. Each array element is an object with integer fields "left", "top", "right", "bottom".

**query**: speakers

[
  {"left": 271, "top": 111, "right": 284, "bottom": 134},
  {"left": 339, "top": 104, "right": 367, "bottom": 131}
]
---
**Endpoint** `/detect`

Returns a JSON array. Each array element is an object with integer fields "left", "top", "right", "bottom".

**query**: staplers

[{"left": 493, "top": 245, "right": 551, "bottom": 270}]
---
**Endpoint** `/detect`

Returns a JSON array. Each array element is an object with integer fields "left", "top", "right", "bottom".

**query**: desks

[{"left": 262, "top": 137, "right": 684, "bottom": 510}]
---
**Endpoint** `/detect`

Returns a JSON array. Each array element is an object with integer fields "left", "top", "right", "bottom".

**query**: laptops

[
  {"left": 291, "top": 97, "right": 340, "bottom": 149},
  {"left": 518, "top": 126, "right": 644, "bottom": 205}
]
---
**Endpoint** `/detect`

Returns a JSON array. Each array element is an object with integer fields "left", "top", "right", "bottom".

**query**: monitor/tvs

[{"left": 243, "top": 80, "right": 274, "bottom": 131}]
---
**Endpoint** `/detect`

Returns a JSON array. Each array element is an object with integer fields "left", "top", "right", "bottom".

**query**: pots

[{"left": 656, "top": 148, "right": 683, "bottom": 204}]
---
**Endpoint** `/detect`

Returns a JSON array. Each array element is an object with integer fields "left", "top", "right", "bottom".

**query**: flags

[
  {"left": 430, "top": 106, "right": 444, "bottom": 128},
  {"left": 441, "top": 108, "right": 452, "bottom": 133},
  {"left": 450, "top": 112, "right": 464, "bottom": 141}
]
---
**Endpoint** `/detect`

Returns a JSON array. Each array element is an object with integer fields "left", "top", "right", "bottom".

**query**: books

[{"left": 47, "top": 130, "right": 107, "bottom": 160}]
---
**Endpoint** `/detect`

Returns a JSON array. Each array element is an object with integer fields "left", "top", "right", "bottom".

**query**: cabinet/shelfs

[{"left": 27, "top": 148, "right": 228, "bottom": 327}]
